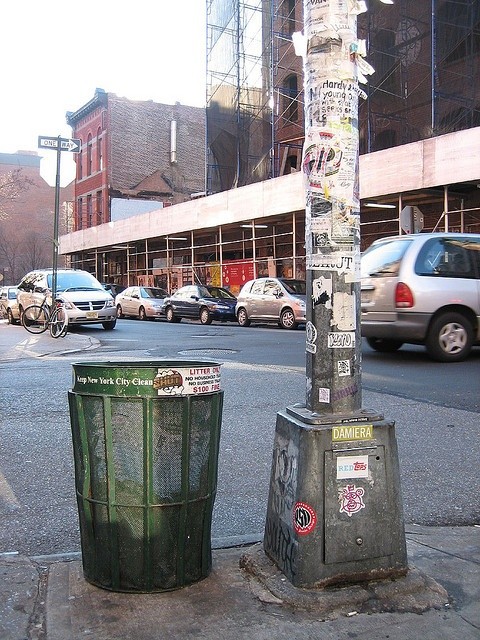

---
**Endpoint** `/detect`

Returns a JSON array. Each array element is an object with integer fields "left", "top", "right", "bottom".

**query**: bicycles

[{"left": 22, "top": 294, "right": 69, "bottom": 338}]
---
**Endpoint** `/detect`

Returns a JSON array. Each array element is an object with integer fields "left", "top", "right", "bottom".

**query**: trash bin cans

[{"left": 66, "top": 361, "right": 223, "bottom": 593}]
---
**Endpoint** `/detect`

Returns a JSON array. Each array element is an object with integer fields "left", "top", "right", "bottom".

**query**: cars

[
  {"left": 164, "top": 284, "right": 238, "bottom": 324},
  {"left": 115, "top": 285, "right": 173, "bottom": 323},
  {"left": 100, "top": 282, "right": 125, "bottom": 297},
  {"left": 234, "top": 276, "right": 307, "bottom": 330},
  {"left": 1, "top": 285, "right": 23, "bottom": 325}
]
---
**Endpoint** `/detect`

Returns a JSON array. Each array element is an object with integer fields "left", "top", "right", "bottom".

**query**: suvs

[
  {"left": 358, "top": 232, "right": 479, "bottom": 361},
  {"left": 17, "top": 266, "right": 117, "bottom": 332}
]
